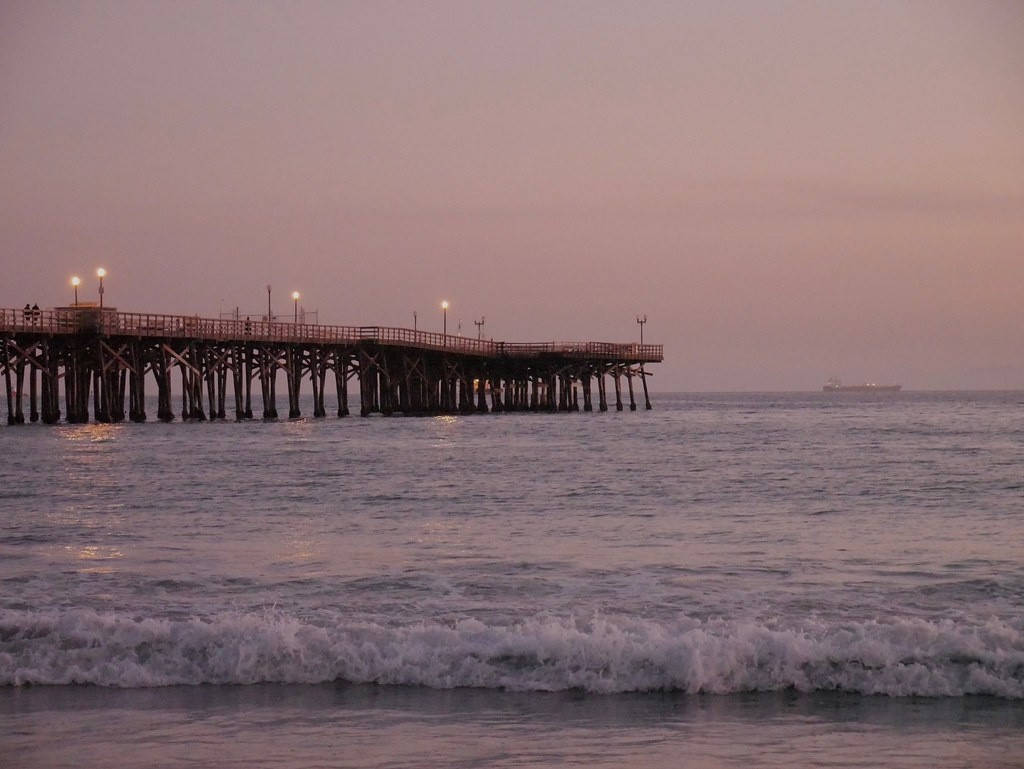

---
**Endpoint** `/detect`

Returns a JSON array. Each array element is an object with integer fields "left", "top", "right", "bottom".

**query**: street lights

[
  {"left": 293, "top": 292, "right": 300, "bottom": 322},
  {"left": 413, "top": 311, "right": 417, "bottom": 332},
  {"left": 72, "top": 277, "right": 82, "bottom": 308},
  {"left": 267, "top": 286, "right": 273, "bottom": 322},
  {"left": 442, "top": 301, "right": 448, "bottom": 348},
  {"left": 96, "top": 268, "right": 106, "bottom": 312}
]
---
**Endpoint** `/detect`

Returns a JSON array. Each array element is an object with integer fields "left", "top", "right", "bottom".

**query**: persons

[
  {"left": 24, "top": 304, "right": 31, "bottom": 326},
  {"left": 31, "top": 303, "right": 40, "bottom": 326},
  {"left": 244, "top": 317, "right": 251, "bottom": 336}
]
